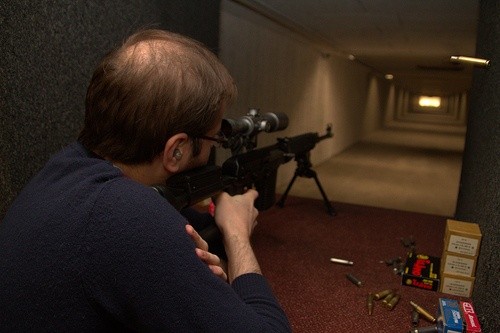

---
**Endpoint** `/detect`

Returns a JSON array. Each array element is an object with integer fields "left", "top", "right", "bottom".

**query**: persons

[{"left": 0, "top": 29, "right": 290, "bottom": 333}]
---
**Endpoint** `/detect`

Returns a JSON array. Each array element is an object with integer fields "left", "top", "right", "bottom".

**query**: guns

[{"left": 150, "top": 109, "right": 336, "bottom": 218}]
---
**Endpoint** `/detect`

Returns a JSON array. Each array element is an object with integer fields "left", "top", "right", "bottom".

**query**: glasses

[{"left": 198, "top": 130, "right": 225, "bottom": 144}]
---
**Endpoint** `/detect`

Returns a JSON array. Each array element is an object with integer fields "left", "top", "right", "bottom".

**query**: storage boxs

[
  {"left": 443, "top": 219, "right": 481, "bottom": 256},
  {"left": 437, "top": 298, "right": 481, "bottom": 333},
  {"left": 441, "top": 250, "right": 478, "bottom": 278},
  {"left": 401, "top": 255, "right": 441, "bottom": 292},
  {"left": 440, "top": 274, "right": 475, "bottom": 298}
]
